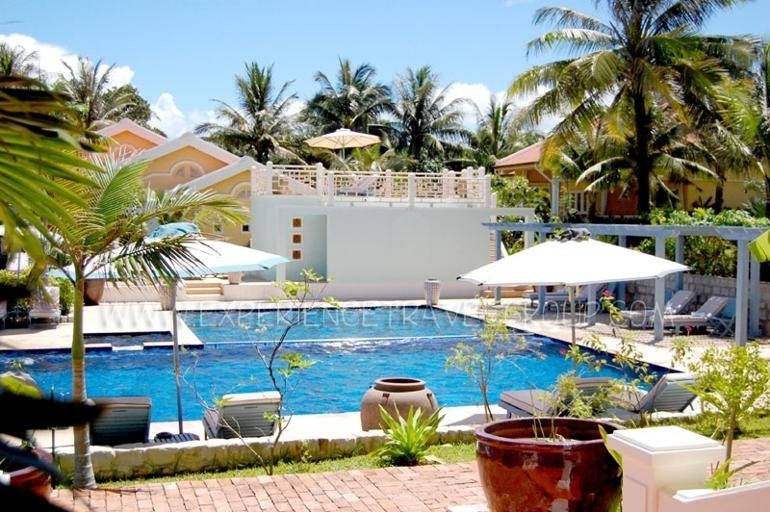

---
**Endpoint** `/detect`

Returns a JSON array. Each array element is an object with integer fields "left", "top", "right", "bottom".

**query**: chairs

[
  {"left": 619, "top": 288, "right": 730, "bottom": 338},
  {"left": 498, "top": 372, "right": 707, "bottom": 420},
  {"left": 29, "top": 287, "right": 61, "bottom": 330},
  {"left": 527, "top": 283, "right": 608, "bottom": 311},
  {"left": 84, "top": 390, "right": 282, "bottom": 446}
]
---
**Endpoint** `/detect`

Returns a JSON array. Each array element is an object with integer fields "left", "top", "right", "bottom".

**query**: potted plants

[{"left": 473, "top": 349, "right": 624, "bottom": 512}]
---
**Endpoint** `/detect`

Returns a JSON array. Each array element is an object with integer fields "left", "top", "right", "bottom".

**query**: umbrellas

[
  {"left": 302, "top": 127, "right": 382, "bottom": 161},
  {"left": 46, "top": 226, "right": 292, "bottom": 435},
  {"left": 457, "top": 228, "right": 697, "bottom": 378}
]
